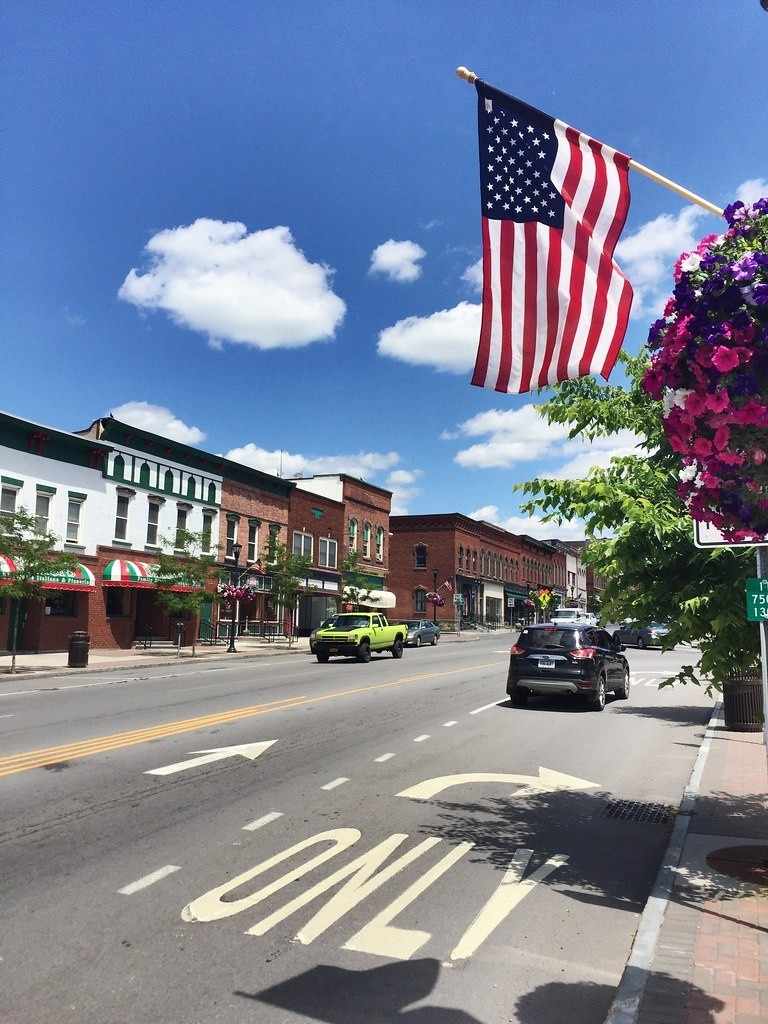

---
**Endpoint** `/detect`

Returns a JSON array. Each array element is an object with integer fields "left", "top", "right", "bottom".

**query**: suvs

[
  {"left": 506, "top": 623, "right": 630, "bottom": 712},
  {"left": 549, "top": 608, "right": 587, "bottom": 625}
]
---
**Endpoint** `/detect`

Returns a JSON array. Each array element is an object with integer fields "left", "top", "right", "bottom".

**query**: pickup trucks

[{"left": 316, "top": 612, "right": 409, "bottom": 663}]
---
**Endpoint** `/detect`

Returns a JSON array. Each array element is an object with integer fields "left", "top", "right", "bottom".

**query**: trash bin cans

[
  {"left": 67, "top": 630, "right": 92, "bottom": 668},
  {"left": 722, "top": 649, "right": 764, "bottom": 732},
  {"left": 516, "top": 617, "right": 525, "bottom": 632}
]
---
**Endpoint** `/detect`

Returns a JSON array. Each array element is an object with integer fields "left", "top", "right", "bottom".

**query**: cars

[
  {"left": 585, "top": 612, "right": 606, "bottom": 628},
  {"left": 309, "top": 614, "right": 369, "bottom": 654},
  {"left": 618, "top": 615, "right": 633, "bottom": 626},
  {"left": 613, "top": 620, "right": 675, "bottom": 650},
  {"left": 395, "top": 618, "right": 441, "bottom": 648}
]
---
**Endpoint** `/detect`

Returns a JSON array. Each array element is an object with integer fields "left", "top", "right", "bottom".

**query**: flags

[
  {"left": 468, "top": 75, "right": 634, "bottom": 393},
  {"left": 250, "top": 559, "right": 267, "bottom": 574},
  {"left": 535, "top": 588, "right": 549, "bottom": 610},
  {"left": 445, "top": 578, "right": 453, "bottom": 590}
]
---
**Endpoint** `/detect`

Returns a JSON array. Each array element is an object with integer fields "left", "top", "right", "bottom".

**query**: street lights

[
  {"left": 226, "top": 539, "right": 243, "bottom": 653},
  {"left": 525, "top": 578, "right": 531, "bottom": 625},
  {"left": 432, "top": 566, "right": 439, "bottom": 626}
]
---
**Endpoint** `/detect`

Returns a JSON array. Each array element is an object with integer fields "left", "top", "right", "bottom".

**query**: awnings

[
  {"left": 343, "top": 587, "right": 397, "bottom": 607},
  {"left": 0, "top": 551, "right": 206, "bottom": 594}
]
